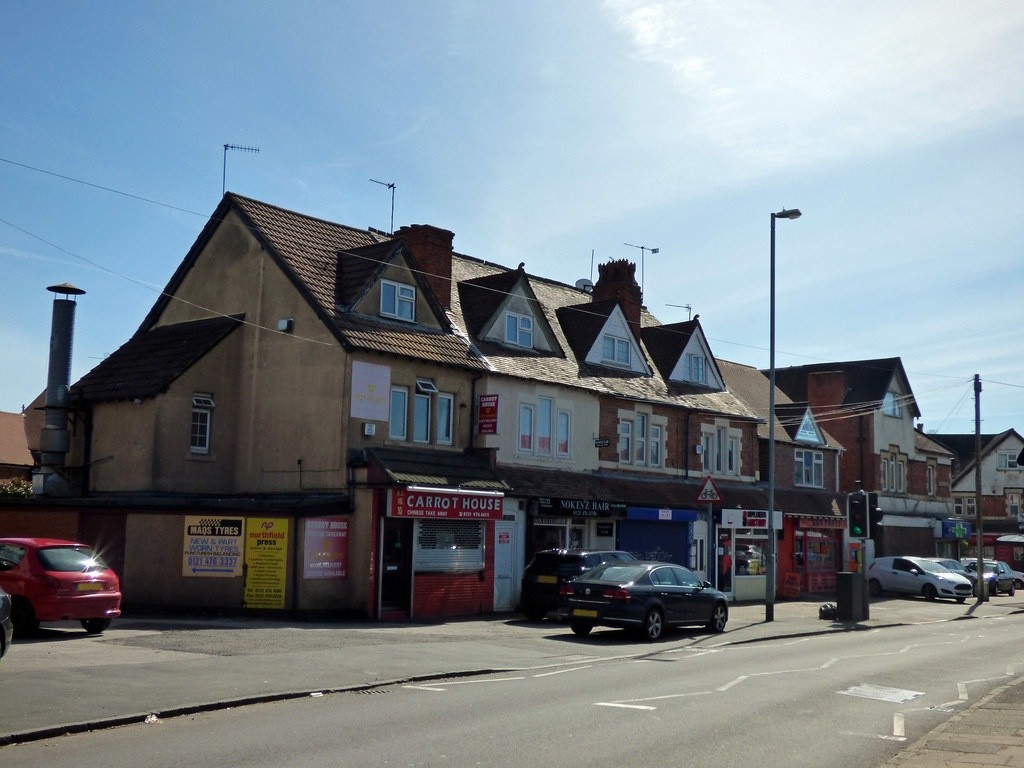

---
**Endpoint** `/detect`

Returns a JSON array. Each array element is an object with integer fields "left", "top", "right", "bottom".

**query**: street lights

[{"left": 763, "top": 209, "right": 803, "bottom": 622}]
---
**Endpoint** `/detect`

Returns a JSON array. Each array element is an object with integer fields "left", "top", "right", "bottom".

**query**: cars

[
  {"left": 959, "top": 558, "right": 1024, "bottom": 597},
  {"left": 735, "top": 544, "right": 765, "bottom": 563},
  {"left": 866, "top": 555, "right": 973, "bottom": 603},
  {"left": 0, "top": 587, "right": 14, "bottom": 660},
  {"left": 926, "top": 557, "right": 977, "bottom": 596},
  {"left": 0, "top": 537, "right": 122, "bottom": 639},
  {"left": 559, "top": 559, "right": 729, "bottom": 641}
]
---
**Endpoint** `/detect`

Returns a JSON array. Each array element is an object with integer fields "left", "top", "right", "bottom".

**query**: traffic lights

[
  {"left": 867, "top": 492, "right": 884, "bottom": 539},
  {"left": 848, "top": 494, "right": 869, "bottom": 539}
]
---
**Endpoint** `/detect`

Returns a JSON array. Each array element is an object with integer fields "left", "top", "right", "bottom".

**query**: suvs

[{"left": 519, "top": 548, "right": 638, "bottom": 624}]
